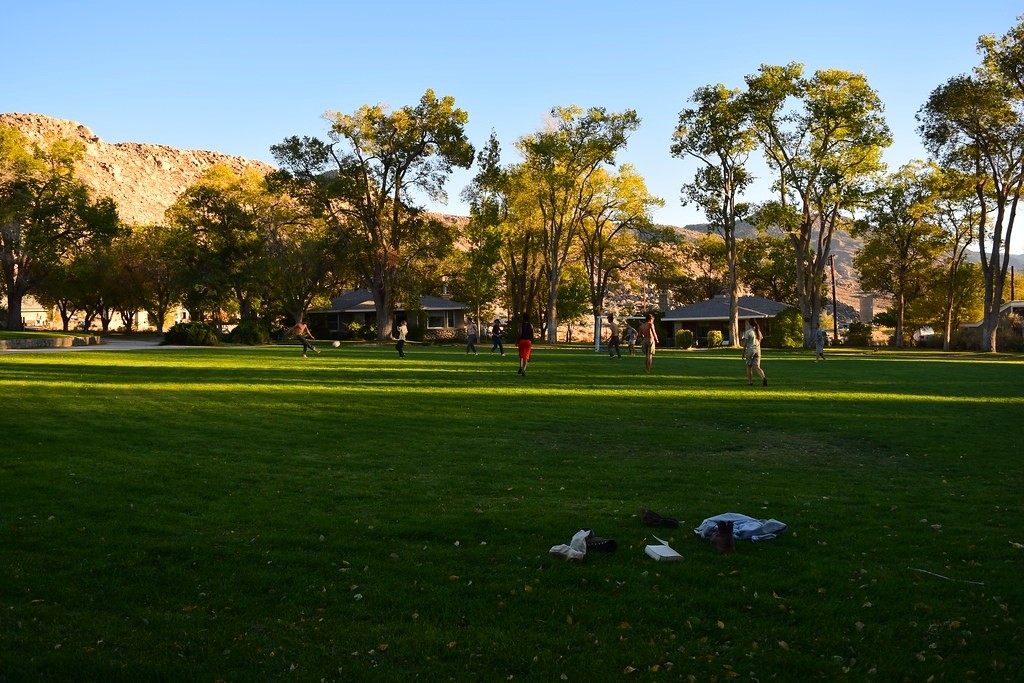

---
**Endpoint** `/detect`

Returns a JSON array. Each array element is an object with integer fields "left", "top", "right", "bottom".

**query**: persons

[
  {"left": 624, "top": 323, "right": 638, "bottom": 356},
  {"left": 639, "top": 314, "right": 659, "bottom": 374},
  {"left": 284, "top": 318, "right": 321, "bottom": 358},
  {"left": 517, "top": 313, "right": 533, "bottom": 375},
  {"left": 465, "top": 317, "right": 478, "bottom": 355},
  {"left": 395, "top": 321, "right": 408, "bottom": 358},
  {"left": 741, "top": 317, "right": 768, "bottom": 387},
  {"left": 638, "top": 320, "right": 646, "bottom": 354},
  {"left": 490, "top": 319, "right": 505, "bottom": 357},
  {"left": 811, "top": 323, "right": 825, "bottom": 363},
  {"left": 602, "top": 315, "right": 622, "bottom": 360}
]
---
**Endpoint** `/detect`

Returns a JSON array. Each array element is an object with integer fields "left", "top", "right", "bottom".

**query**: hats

[{"left": 494, "top": 319, "right": 500, "bottom": 324}]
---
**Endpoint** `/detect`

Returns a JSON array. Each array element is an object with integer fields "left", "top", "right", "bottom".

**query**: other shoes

[
  {"left": 814, "top": 361, "right": 818, "bottom": 364},
  {"left": 302, "top": 354, "right": 308, "bottom": 359},
  {"left": 518, "top": 368, "right": 526, "bottom": 376},
  {"left": 639, "top": 507, "right": 681, "bottom": 529},
  {"left": 823, "top": 358, "right": 825, "bottom": 361},
  {"left": 475, "top": 352, "right": 478, "bottom": 356},
  {"left": 709, "top": 521, "right": 735, "bottom": 552},
  {"left": 747, "top": 383, "right": 753, "bottom": 386},
  {"left": 490, "top": 350, "right": 493, "bottom": 355},
  {"left": 314, "top": 349, "right": 321, "bottom": 355},
  {"left": 610, "top": 356, "right": 615, "bottom": 360},
  {"left": 464, "top": 352, "right": 468, "bottom": 357},
  {"left": 763, "top": 377, "right": 768, "bottom": 387},
  {"left": 501, "top": 353, "right": 505, "bottom": 357},
  {"left": 644, "top": 368, "right": 651, "bottom": 374},
  {"left": 400, "top": 354, "right": 406, "bottom": 359},
  {"left": 616, "top": 358, "right": 622, "bottom": 361}
]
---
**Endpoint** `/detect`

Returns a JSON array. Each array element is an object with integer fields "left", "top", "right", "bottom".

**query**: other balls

[{"left": 332, "top": 340, "right": 341, "bottom": 347}]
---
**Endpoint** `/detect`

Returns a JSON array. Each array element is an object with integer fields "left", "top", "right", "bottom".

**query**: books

[{"left": 644, "top": 533, "right": 686, "bottom": 562}]
